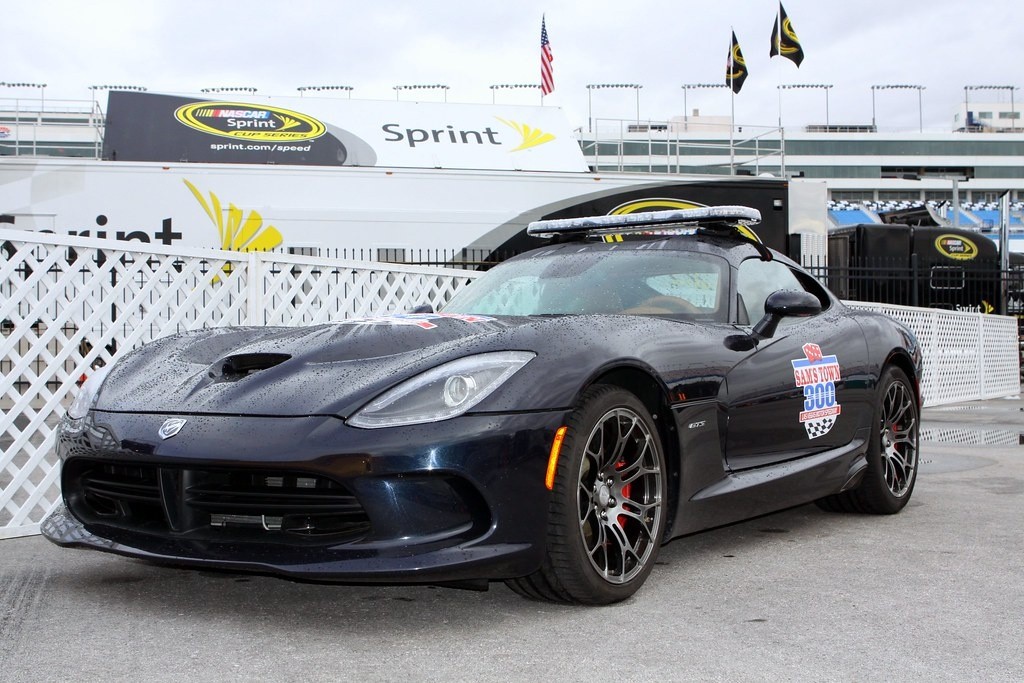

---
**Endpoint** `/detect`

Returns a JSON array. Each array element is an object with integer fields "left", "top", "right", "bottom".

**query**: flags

[
  {"left": 769, "top": 3, "right": 804, "bottom": 69},
  {"left": 725, "top": 32, "right": 749, "bottom": 94},
  {"left": 540, "top": 14, "right": 555, "bottom": 96}
]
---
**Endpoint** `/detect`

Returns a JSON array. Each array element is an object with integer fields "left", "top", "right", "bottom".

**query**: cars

[{"left": 39, "top": 205, "right": 924, "bottom": 607}]
